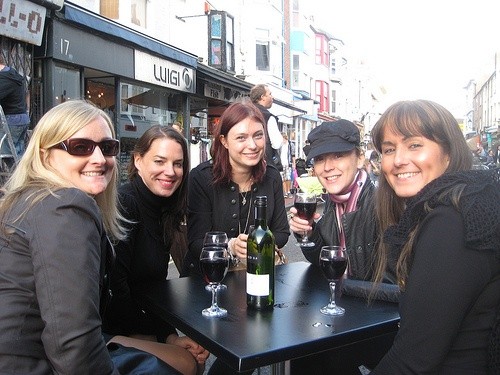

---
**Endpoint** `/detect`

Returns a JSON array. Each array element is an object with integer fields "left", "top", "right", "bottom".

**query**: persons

[
  {"left": 0, "top": 55, "right": 31, "bottom": 174},
  {"left": 0, "top": 98, "right": 185, "bottom": 375},
  {"left": 102, "top": 125, "right": 210, "bottom": 375},
  {"left": 187, "top": 100, "right": 290, "bottom": 373},
  {"left": 371, "top": 99, "right": 500, "bottom": 375},
  {"left": 289, "top": 119, "right": 408, "bottom": 375},
  {"left": 281, "top": 130, "right": 311, "bottom": 198},
  {"left": 172, "top": 122, "right": 184, "bottom": 134},
  {"left": 249, "top": 84, "right": 284, "bottom": 172}
]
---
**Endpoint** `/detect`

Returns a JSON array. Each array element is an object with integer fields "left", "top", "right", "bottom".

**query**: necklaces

[{"left": 239, "top": 183, "right": 249, "bottom": 206}]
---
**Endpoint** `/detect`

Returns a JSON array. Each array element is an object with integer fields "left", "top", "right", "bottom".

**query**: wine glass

[
  {"left": 200, "top": 246, "right": 229, "bottom": 319},
  {"left": 295, "top": 193, "right": 317, "bottom": 247},
  {"left": 318, "top": 245, "right": 348, "bottom": 316},
  {"left": 203, "top": 231, "right": 229, "bottom": 291}
]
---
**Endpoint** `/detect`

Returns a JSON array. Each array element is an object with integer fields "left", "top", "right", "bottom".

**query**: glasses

[{"left": 48, "top": 138, "right": 120, "bottom": 156}]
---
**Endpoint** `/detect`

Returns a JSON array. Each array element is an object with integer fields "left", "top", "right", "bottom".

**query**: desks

[{"left": 144, "top": 260, "right": 401, "bottom": 375}]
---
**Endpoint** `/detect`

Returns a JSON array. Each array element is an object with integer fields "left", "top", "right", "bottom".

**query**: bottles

[{"left": 245, "top": 196, "right": 275, "bottom": 312}]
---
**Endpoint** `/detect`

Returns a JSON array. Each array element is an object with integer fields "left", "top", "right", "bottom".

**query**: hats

[{"left": 307, "top": 119, "right": 360, "bottom": 159}]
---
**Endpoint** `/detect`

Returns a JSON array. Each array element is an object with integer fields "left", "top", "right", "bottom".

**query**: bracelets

[
  {"left": 26, "top": 110, "right": 29, "bottom": 112},
  {"left": 228, "top": 238, "right": 239, "bottom": 260}
]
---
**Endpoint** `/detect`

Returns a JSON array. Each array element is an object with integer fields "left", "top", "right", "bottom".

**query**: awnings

[{"left": 122, "top": 88, "right": 188, "bottom": 113}]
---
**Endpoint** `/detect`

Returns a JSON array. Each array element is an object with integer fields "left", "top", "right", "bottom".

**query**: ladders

[{"left": 0, "top": 106, "right": 19, "bottom": 186}]
